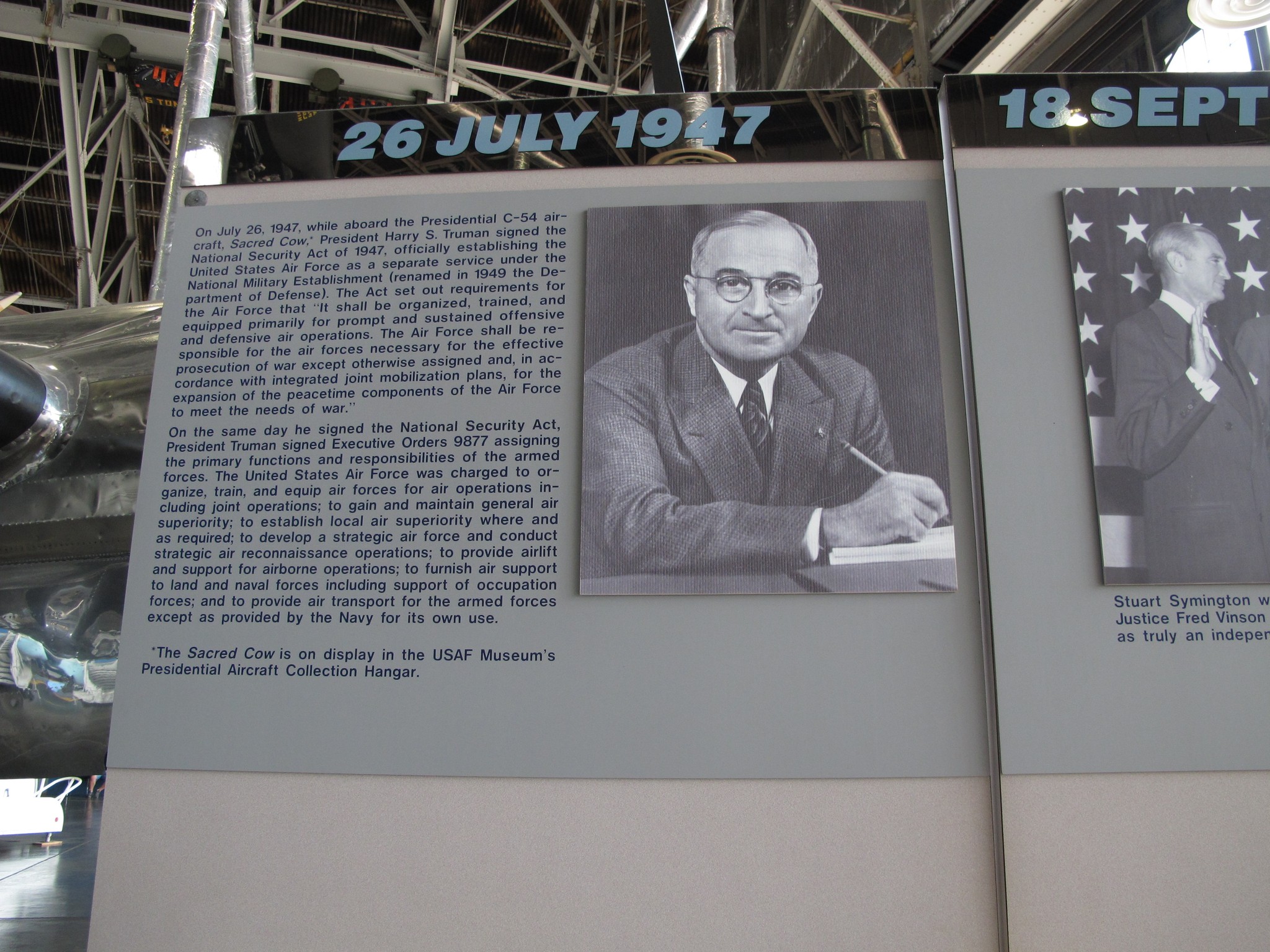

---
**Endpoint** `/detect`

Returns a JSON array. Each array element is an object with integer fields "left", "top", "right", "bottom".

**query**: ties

[
  {"left": 1202, "top": 317, "right": 1237, "bottom": 378},
  {"left": 741, "top": 380, "right": 772, "bottom": 478}
]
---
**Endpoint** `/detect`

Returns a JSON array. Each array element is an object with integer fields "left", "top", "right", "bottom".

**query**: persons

[
  {"left": 1111, "top": 222, "right": 1270, "bottom": 585},
  {"left": 583, "top": 208, "right": 953, "bottom": 582}
]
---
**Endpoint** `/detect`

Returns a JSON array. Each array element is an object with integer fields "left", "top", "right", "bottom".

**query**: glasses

[{"left": 695, "top": 274, "right": 815, "bottom": 305}]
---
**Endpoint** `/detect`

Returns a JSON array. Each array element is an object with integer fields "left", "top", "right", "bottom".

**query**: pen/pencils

[{"left": 840, "top": 440, "right": 952, "bottom": 525}]
